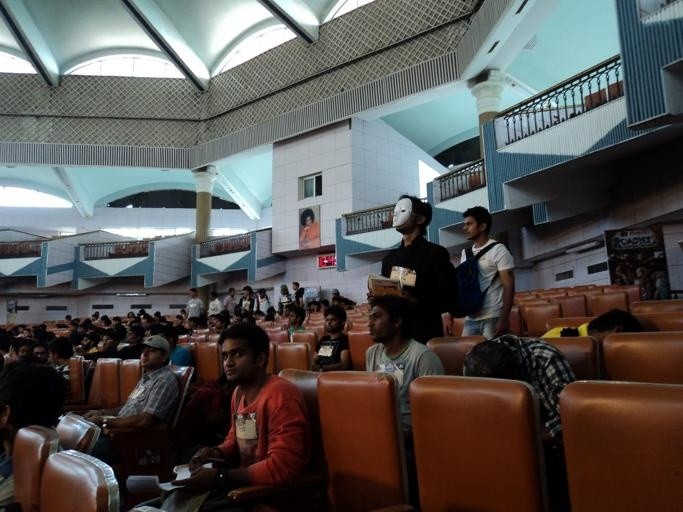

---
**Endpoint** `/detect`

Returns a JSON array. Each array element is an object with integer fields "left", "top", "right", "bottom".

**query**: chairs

[
  {"left": 380, "top": 208, "right": 394, "bottom": 228},
  {"left": 584, "top": 89, "right": 607, "bottom": 112},
  {"left": 0, "top": 240, "right": 43, "bottom": 258},
  {"left": 457, "top": 174, "right": 481, "bottom": 195},
  {"left": 0, "top": 284, "right": 683, "bottom": 512},
  {"left": 107, "top": 240, "right": 147, "bottom": 257},
  {"left": 209, "top": 235, "right": 250, "bottom": 256},
  {"left": 608, "top": 80, "right": 624, "bottom": 102}
]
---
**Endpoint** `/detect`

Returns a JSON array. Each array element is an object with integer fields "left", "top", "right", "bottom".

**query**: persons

[
  {"left": 365, "top": 292, "right": 445, "bottom": 511},
  {"left": 83, "top": 334, "right": 179, "bottom": 489},
  {"left": 310, "top": 303, "right": 351, "bottom": 372},
  {"left": 64, "top": 307, "right": 306, "bottom": 362},
  {"left": 2, "top": 323, "right": 74, "bottom": 480},
  {"left": 185, "top": 285, "right": 273, "bottom": 320},
  {"left": 443, "top": 206, "right": 516, "bottom": 340},
  {"left": 267, "top": 281, "right": 357, "bottom": 333},
  {"left": 463, "top": 334, "right": 576, "bottom": 441},
  {"left": 540, "top": 308, "right": 642, "bottom": 381},
  {"left": 161, "top": 321, "right": 309, "bottom": 510},
  {"left": 635, "top": 266, "right": 669, "bottom": 301},
  {"left": 365, "top": 193, "right": 456, "bottom": 344},
  {"left": 299, "top": 209, "right": 320, "bottom": 249}
]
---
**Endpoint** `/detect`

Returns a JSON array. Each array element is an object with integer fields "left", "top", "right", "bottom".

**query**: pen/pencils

[{"left": 205, "top": 456, "right": 225, "bottom": 462}]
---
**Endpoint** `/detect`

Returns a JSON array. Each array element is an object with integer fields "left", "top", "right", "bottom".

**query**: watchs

[
  {"left": 319, "top": 365, "right": 324, "bottom": 372},
  {"left": 215, "top": 468, "right": 229, "bottom": 480}
]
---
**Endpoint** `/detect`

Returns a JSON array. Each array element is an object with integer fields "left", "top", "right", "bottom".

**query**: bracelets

[{"left": 102, "top": 419, "right": 107, "bottom": 428}]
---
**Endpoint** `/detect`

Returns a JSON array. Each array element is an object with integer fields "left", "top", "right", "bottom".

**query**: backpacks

[{"left": 452, "top": 242, "right": 501, "bottom": 318}]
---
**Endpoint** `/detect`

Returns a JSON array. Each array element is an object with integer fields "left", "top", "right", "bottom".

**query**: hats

[{"left": 139, "top": 335, "right": 169, "bottom": 353}]
